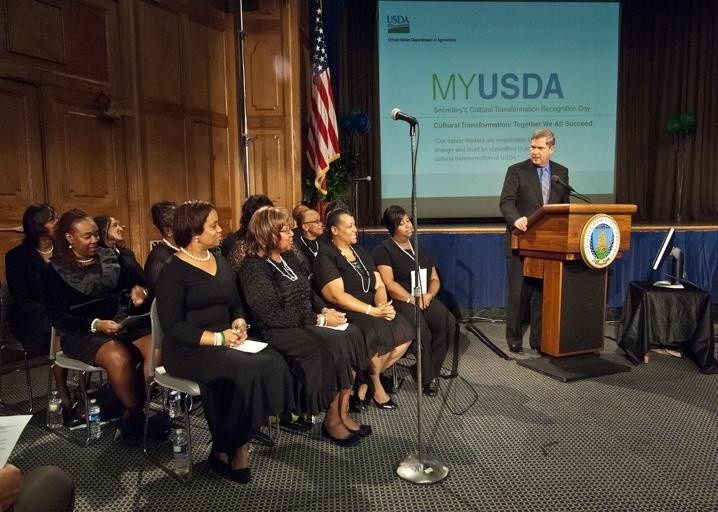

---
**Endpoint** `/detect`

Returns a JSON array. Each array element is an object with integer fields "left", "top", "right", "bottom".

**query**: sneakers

[{"left": 62, "top": 393, "right": 80, "bottom": 426}]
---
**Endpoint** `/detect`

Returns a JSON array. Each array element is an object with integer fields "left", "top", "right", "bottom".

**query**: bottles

[
  {"left": 169, "top": 389, "right": 182, "bottom": 419},
  {"left": 173, "top": 429, "right": 189, "bottom": 476},
  {"left": 88, "top": 399, "right": 101, "bottom": 439},
  {"left": 49, "top": 390, "right": 64, "bottom": 429}
]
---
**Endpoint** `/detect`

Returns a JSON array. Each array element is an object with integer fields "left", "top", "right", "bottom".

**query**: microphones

[
  {"left": 552, "top": 177, "right": 580, "bottom": 197},
  {"left": 390, "top": 108, "right": 418, "bottom": 125},
  {"left": 355, "top": 175, "right": 373, "bottom": 181}
]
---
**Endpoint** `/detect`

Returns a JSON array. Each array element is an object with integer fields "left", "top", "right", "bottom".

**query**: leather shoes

[
  {"left": 113, "top": 427, "right": 122, "bottom": 442},
  {"left": 509, "top": 344, "right": 525, "bottom": 355},
  {"left": 370, "top": 391, "right": 398, "bottom": 410},
  {"left": 409, "top": 364, "right": 417, "bottom": 383},
  {"left": 349, "top": 388, "right": 371, "bottom": 413},
  {"left": 529, "top": 340, "right": 541, "bottom": 353},
  {"left": 320, "top": 418, "right": 360, "bottom": 447},
  {"left": 208, "top": 450, "right": 251, "bottom": 484},
  {"left": 423, "top": 381, "right": 438, "bottom": 396},
  {"left": 279, "top": 408, "right": 314, "bottom": 433},
  {"left": 345, "top": 423, "right": 372, "bottom": 437}
]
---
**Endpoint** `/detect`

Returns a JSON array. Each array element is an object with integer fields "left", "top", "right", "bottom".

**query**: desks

[{"left": 618, "top": 281, "right": 711, "bottom": 368}]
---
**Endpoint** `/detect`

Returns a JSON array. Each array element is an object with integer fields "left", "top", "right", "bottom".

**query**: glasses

[
  {"left": 304, "top": 219, "right": 319, "bottom": 224},
  {"left": 279, "top": 228, "right": 290, "bottom": 235}
]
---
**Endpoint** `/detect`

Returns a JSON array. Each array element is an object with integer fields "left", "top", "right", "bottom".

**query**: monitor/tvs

[{"left": 652, "top": 227, "right": 688, "bottom": 290}]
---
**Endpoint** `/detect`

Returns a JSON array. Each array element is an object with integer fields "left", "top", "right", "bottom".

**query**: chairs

[
  {"left": 142, "top": 297, "right": 203, "bottom": 484},
  {"left": 46, "top": 326, "right": 106, "bottom": 447},
  {"left": 0, "top": 285, "right": 34, "bottom": 415}
]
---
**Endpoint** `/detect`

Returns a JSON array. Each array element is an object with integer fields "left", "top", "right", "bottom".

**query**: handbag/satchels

[{"left": 94, "top": 382, "right": 126, "bottom": 422}]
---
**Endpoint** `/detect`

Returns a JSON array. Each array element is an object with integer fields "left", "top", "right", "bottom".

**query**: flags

[{"left": 306, "top": 0, "right": 343, "bottom": 197}]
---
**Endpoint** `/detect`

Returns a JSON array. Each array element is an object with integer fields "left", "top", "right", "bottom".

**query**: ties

[{"left": 540, "top": 167, "right": 551, "bottom": 205}]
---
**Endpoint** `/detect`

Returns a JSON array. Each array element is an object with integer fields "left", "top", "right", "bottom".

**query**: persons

[
  {"left": 0, "top": 192, "right": 458, "bottom": 485},
  {"left": 497, "top": 130, "right": 570, "bottom": 357},
  {"left": 0, "top": 462, "right": 76, "bottom": 510}
]
